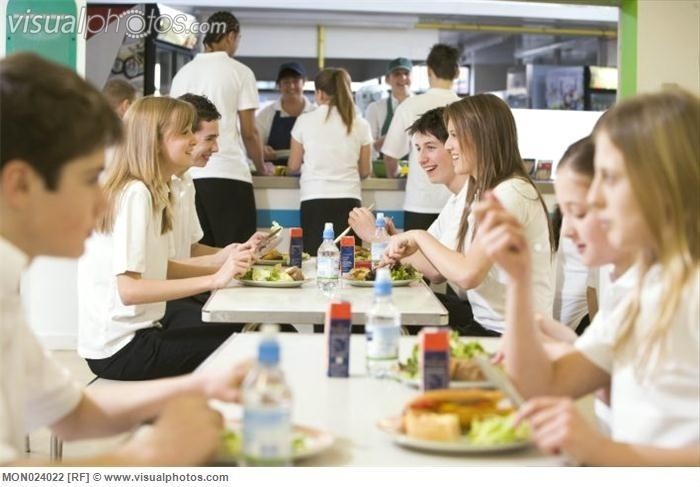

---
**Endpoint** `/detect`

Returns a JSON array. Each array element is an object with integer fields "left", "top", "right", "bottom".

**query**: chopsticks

[{"left": 333, "top": 202, "right": 376, "bottom": 243}]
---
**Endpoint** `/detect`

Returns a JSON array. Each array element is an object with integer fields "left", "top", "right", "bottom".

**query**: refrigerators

[{"left": 0, "top": 0, "right": 201, "bottom": 108}]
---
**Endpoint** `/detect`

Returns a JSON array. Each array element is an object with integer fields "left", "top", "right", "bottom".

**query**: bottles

[
  {"left": 362, "top": 267, "right": 405, "bottom": 374},
  {"left": 236, "top": 336, "right": 300, "bottom": 467},
  {"left": 314, "top": 221, "right": 341, "bottom": 293},
  {"left": 370, "top": 213, "right": 394, "bottom": 276}
]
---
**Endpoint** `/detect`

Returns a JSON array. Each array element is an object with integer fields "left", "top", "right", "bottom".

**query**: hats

[
  {"left": 386, "top": 58, "right": 412, "bottom": 75},
  {"left": 203, "top": 13, "right": 240, "bottom": 44},
  {"left": 278, "top": 62, "right": 305, "bottom": 77}
]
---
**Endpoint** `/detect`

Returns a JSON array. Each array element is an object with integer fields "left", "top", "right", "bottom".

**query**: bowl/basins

[{"left": 370, "top": 159, "right": 409, "bottom": 178}]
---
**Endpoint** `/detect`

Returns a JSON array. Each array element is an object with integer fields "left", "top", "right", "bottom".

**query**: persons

[{"left": 1, "top": 13, "right": 699, "bottom": 468}]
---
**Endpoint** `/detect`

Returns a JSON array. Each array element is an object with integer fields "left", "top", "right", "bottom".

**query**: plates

[
  {"left": 231, "top": 266, "right": 310, "bottom": 289},
  {"left": 190, "top": 406, "right": 339, "bottom": 464},
  {"left": 368, "top": 392, "right": 539, "bottom": 455},
  {"left": 343, "top": 268, "right": 426, "bottom": 289},
  {"left": 239, "top": 243, "right": 313, "bottom": 270}
]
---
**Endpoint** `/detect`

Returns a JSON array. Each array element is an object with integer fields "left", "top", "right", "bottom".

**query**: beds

[{"left": 201, "top": 254, "right": 450, "bottom": 332}]
